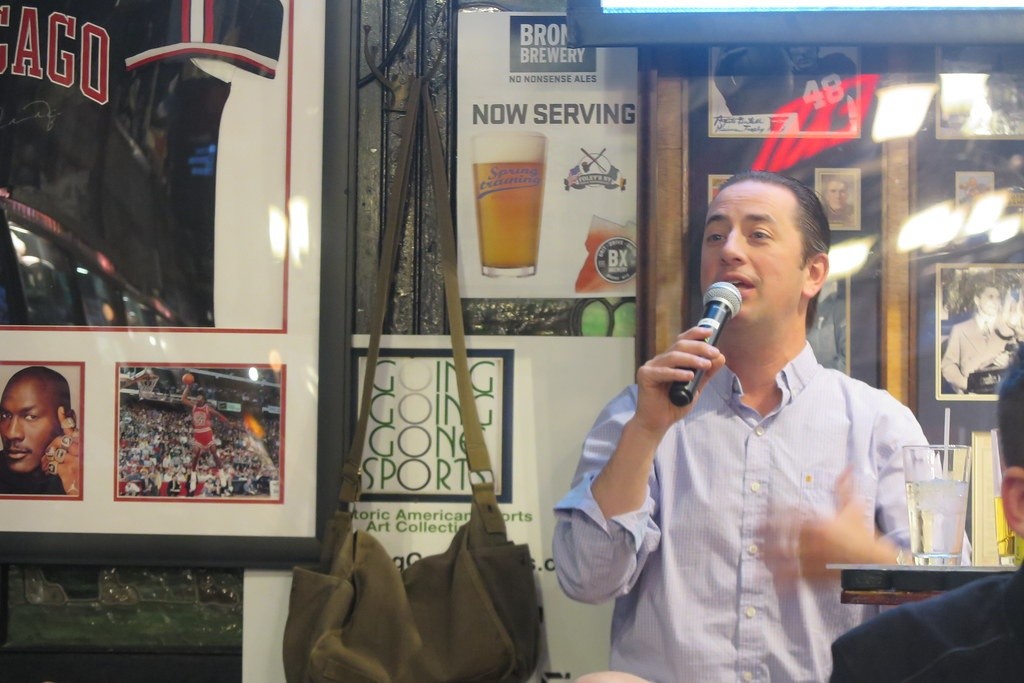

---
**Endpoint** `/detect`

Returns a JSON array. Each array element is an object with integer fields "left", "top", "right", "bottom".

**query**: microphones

[{"left": 669, "top": 281, "right": 742, "bottom": 407}]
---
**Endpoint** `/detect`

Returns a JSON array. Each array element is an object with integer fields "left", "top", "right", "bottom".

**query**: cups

[
  {"left": 473, "top": 133, "right": 547, "bottom": 278},
  {"left": 991, "top": 428, "right": 1024, "bottom": 567},
  {"left": 902, "top": 445, "right": 970, "bottom": 566}
]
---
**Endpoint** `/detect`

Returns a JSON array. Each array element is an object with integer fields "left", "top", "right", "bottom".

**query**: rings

[{"left": 43, "top": 411, "right": 79, "bottom": 474}]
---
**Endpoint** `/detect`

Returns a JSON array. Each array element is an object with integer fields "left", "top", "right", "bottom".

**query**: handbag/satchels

[{"left": 284, "top": 481, "right": 539, "bottom": 683}]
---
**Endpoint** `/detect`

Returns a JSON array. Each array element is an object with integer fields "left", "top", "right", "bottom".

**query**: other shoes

[
  {"left": 189, "top": 473, "right": 197, "bottom": 491},
  {"left": 219, "top": 473, "right": 227, "bottom": 487}
]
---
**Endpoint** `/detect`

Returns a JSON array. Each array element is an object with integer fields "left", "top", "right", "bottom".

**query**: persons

[
  {"left": 117, "top": 408, "right": 280, "bottom": 498},
  {"left": 0, "top": 366, "right": 80, "bottom": 494},
  {"left": 823, "top": 178, "right": 852, "bottom": 223},
  {"left": 552, "top": 170, "right": 971, "bottom": 683},
  {"left": 180, "top": 383, "right": 236, "bottom": 492},
  {"left": 942, "top": 284, "right": 1018, "bottom": 392},
  {"left": 829, "top": 349, "right": 1024, "bottom": 683}
]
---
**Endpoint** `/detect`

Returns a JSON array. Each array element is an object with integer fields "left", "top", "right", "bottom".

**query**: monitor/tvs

[{"left": 565, "top": 1, "right": 1024, "bottom": 49}]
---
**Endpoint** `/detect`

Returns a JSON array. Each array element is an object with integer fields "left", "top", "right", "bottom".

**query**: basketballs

[{"left": 182, "top": 373, "right": 194, "bottom": 384}]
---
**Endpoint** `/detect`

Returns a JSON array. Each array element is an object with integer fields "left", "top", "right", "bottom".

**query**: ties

[{"left": 983, "top": 320, "right": 991, "bottom": 343}]
